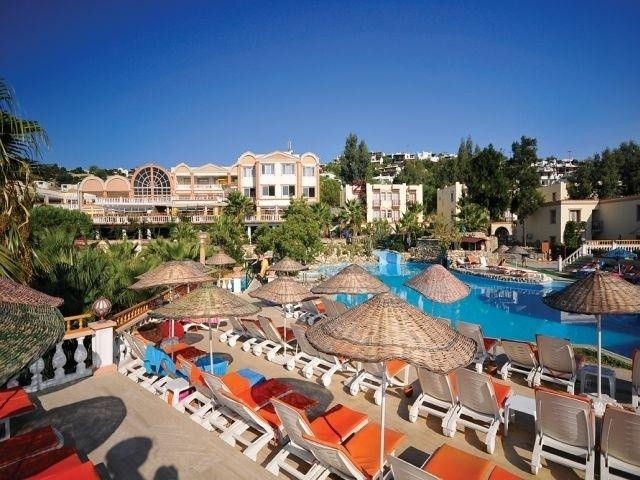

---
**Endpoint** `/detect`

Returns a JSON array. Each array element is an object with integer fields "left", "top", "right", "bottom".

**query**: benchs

[{"left": 0, "top": 387, "right": 37, "bottom": 443}]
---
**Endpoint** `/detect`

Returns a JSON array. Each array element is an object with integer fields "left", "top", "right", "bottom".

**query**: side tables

[
  {"left": 504, "top": 393, "right": 537, "bottom": 437},
  {"left": 581, "top": 365, "right": 617, "bottom": 399}
]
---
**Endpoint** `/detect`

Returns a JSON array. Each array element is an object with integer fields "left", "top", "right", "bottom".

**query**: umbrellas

[
  {"left": 599, "top": 246, "right": 637, "bottom": 273},
  {"left": 311, "top": 263, "right": 391, "bottom": 308},
  {"left": 402, "top": 263, "right": 472, "bottom": 317},
  {"left": 125, "top": 249, "right": 323, "bottom": 412},
  {"left": 541, "top": 267, "right": 640, "bottom": 397},
  {"left": 0, "top": 301, "right": 66, "bottom": 389},
  {"left": 505, "top": 245, "right": 529, "bottom": 268},
  {"left": 304, "top": 292, "right": 478, "bottom": 480},
  {"left": 492, "top": 243, "right": 510, "bottom": 265},
  {"left": 0, "top": 275, "right": 64, "bottom": 309}
]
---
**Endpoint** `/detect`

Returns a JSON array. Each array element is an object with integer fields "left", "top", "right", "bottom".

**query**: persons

[
  {"left": 498, "top": 258, "right": 506, "bottom": 266},
  {"left": 457, "top": 257, "right": 472, "bottom": 269},
  {"left": 520, "top": 248, "right": 528, "bottom": 268}
]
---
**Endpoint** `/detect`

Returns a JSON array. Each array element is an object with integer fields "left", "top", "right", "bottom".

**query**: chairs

[
  {"left": 0, "top": 425, "right": 65, "bottom": 480},
  {"left": 500, "top": 339, "right": 539, "bottom": 388},
  {"left": 531, "top": 385, "right": 599, "bottom": 480},
  {"left": 117, "top": 315, "right": 459, "bottom": 436},
  {"left": 447, "top": 367, "right": 513, "bottom": 454},
  {"left": 387, "top": 443, "right": 494, "bottom": 480},
  {"left": 485, "top": 462, "right": 525, "bottom": 480},
  {"left": 25, "top": 454, "right": 101, "bottom": 480},
  {"left": 266, "top": 396, "right": 369, "bottom": 480},
  {"left": 302, "top": 420, "right": 406, "bottom": 480},
  {"left": 457, "top": 256, "right": 546, "bottom": 278},
  {"left": 533, "top": 334, "right": 587, "bottom": 395},
  {"left": 632, "top": 349, "right": 640, "bottom": 408},
  {"left": 217, "top": 388, "right": 319, "bottom": 463},
  {"left": 456, "top": 320, "right": 501, "bottom": 374},
  {"left": 599, "top": 404, "right": 640, "bottom": 480}
]
